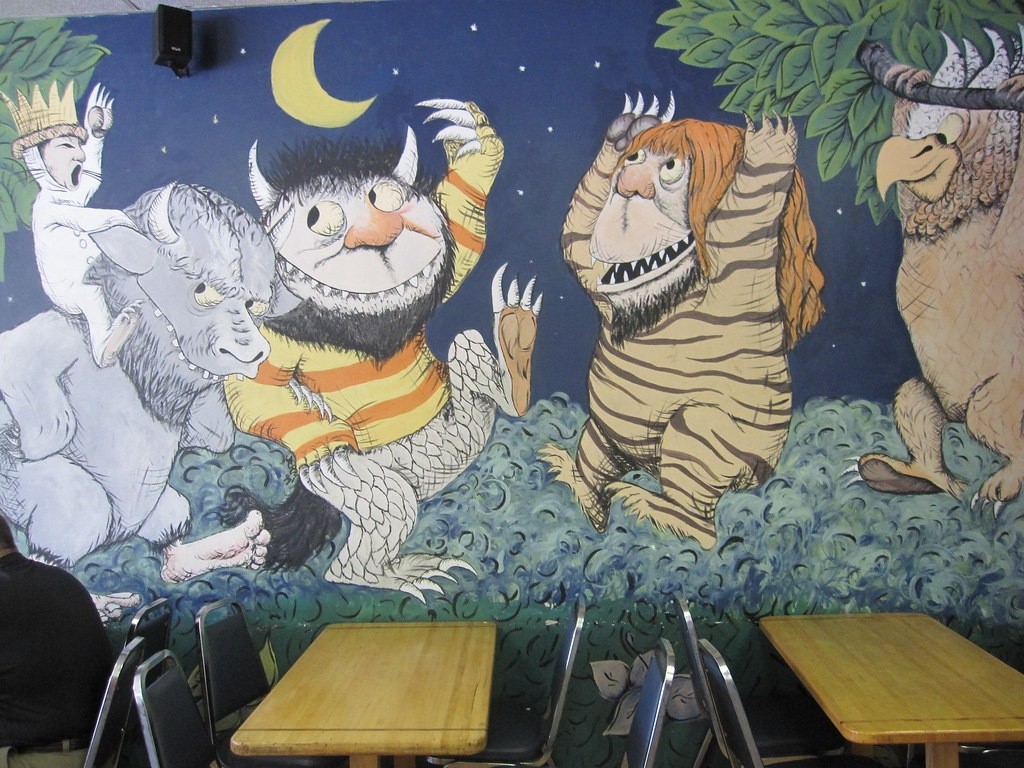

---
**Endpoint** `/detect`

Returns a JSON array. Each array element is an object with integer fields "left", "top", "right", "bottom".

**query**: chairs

[
  {"left": 195, "top": 598, "right": 324, "bottom": 768},
  {"left": 83, "top": 635, "right": 146, "bottom": 767},
  {"left": 698, "top": 637, "right": 887, "bottom": 768},
  {"left": 427, "top": 591, "right": 586, "bottom": 768},
  {"left": 131, "top": 648, "right": 223, "bottom": 768},
  {"left": 618, "top": 637, "right": 676, "bottom": 768},
  {"left": 673, "top": 589, "right": 843, "bottom": 768},
  {"left": 123, "top": 596, "right": 173, "bottom": 691}
]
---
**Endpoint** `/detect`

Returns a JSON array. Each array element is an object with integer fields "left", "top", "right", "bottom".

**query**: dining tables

[
  {"left": 230, "top": 621, "right": 498, "bottom": 768},
  {"left": 759, "top": 610, "right": 1024, "bottom": 768}
]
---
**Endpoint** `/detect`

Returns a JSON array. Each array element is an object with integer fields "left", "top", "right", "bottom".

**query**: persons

[{"left": 0, "top": 512, "right": 111, "bottom": 750}]
